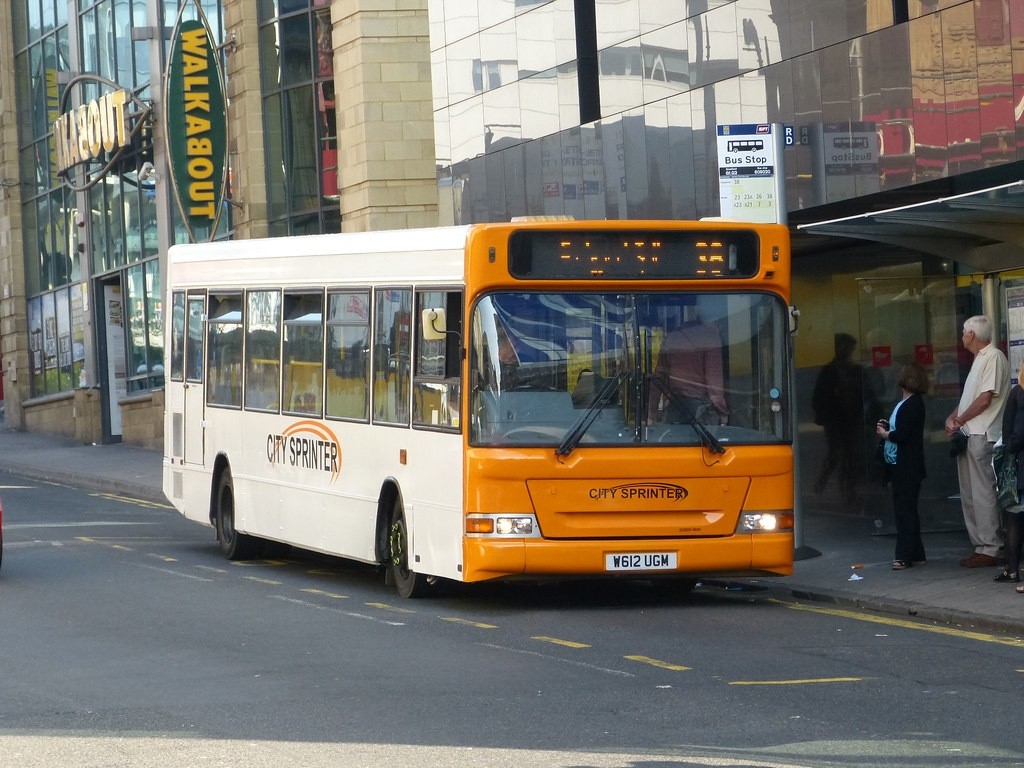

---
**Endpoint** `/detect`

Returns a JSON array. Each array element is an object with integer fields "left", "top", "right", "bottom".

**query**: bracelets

[{"left": 956, "top": 418, "right": 964, "bottom": 426}]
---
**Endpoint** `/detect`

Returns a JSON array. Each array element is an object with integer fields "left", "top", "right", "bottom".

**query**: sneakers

[{"left": 994, "top": 569, "right": 1019, "bottom": 582}]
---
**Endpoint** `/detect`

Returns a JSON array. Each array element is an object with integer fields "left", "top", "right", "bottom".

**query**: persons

[
  {"left": 875, "top": 362, "right": 932, "bottom": 570},
  {"left": 944, "top": 315, "right": 1013, "bottom": 568},
  {"left": 812, "top": 331, "right": 865, "bottom": 501},
  {"left": 648, "top": 305, "right": 731, "bottom": 426},
  {"left": 994, "top": 361, "right": 1024, "bottom": 591}
]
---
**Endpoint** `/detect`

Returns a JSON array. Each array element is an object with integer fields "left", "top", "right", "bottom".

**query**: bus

[{"left": 160, "top": 214, "right": 801, "bottom": 600}]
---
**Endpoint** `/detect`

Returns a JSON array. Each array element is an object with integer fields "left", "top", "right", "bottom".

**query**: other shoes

[
  {"left": 911, "top": 561, "right": 925, "bottom": 564},
  {"left": 961, "top": 553, "right": 979, "bottom": 566},
  {"left": 966, "top": 554, "right": 997, "bottom": 567},
  {"left": 893, "top": 560, "right": 910, "bottom": 569},
  {"left": 1017, "top": 584, "right": 1024, "bottom": 592}
]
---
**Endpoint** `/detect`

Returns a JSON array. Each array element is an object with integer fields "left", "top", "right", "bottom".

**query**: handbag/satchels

[
  {"left": 989, "top": 444, "right": 1019, "bottom": 511},
  {"left": 876, "top": 424, "right": 890, "bottom": 460}
]
